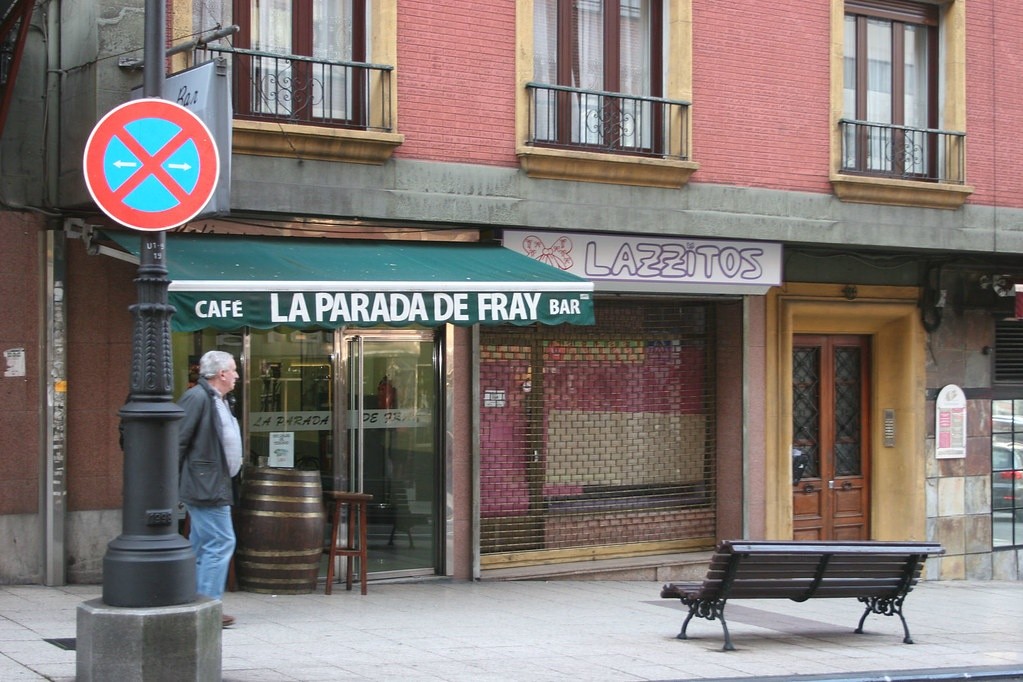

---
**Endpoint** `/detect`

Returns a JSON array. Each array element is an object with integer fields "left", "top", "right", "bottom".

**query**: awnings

[{"left": 104, "top": 228, "right": 596, "bottom": 335}]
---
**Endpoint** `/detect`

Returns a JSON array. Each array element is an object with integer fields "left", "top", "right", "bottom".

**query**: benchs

[
  {"left": 318, "top": 473, "right": 432, "bottom": 549},
  {"left": 660, "top": 539, "right": 945, "bottom": 651}
]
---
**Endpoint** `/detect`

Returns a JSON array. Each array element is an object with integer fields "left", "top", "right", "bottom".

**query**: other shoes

[{"left": 222, "top": 613, "right": 235, "bottom": 626}]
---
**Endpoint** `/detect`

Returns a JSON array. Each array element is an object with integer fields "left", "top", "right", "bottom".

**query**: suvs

[{"left": 992, "top": 440, "right": 1023, "bottom": 522}]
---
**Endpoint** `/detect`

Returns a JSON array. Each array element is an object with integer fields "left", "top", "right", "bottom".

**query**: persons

[{"left": 172, "top": 350, "right": 244, "bottom": 628}]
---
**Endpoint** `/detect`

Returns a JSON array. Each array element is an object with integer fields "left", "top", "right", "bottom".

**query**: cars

[{"left": 992, "top": 414, "right": 1023, "bottom": 442}]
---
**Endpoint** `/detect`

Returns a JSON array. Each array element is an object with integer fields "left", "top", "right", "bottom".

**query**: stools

[{"left": 323, "top": 491, "right": 372, "bottom": 596}]
